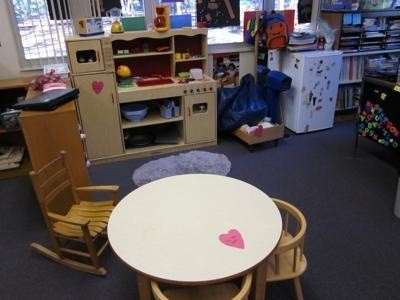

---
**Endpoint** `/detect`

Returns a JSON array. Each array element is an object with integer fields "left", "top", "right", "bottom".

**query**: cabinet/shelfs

[
  {"left": 65, "top": 29, "right": 218, "bottom": 166},
  {"left": 320, "top": 8, "right": 400, "bottom": 115},
  {"left": 0, "top": 75, "right": 95, "bottom": 216}
]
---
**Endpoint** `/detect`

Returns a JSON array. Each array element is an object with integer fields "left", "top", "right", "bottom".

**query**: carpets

[{"left": 132, "top": 151, "right": 231, "bottom": 188}]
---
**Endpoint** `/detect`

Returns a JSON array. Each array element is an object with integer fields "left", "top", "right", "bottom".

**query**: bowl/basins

[{"left": 121, "top": 105, "right": 149, "bottom": 120}]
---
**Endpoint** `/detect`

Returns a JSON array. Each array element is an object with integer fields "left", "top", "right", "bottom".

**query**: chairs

[
  {"left": 252, "top": 197, "right": 309, "bottom": 300},
  {"left": 29, "top": 150, "right": 119, "bottom": 276},
  {"left": 150, "top": 270, "right": 253, "bottom": 300}
]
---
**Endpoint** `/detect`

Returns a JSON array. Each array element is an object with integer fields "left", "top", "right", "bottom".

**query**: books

[
  {"left": 286, "top": 31, "right": 317, "bottom": 52},
  {"left": 335, "top": 12, "right": 399, "bottom": 110},
  {"left": 0, "top": 145, "right": 26, "bottom": 170}
]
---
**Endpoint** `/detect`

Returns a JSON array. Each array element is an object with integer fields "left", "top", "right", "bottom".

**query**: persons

[
  {"left": 213, "top": 57, "right": 227, "bottom": 80},
  {"left": 222, "top": 62, "right": 237, "bottom": 82}
]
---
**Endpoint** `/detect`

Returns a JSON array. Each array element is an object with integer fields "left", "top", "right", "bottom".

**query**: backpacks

[
  {"left": 246, "top": 16, "right": 258, "bottom": 46},
  {"left": 260, "top": 10, "right": 289, "bottom": 49}
]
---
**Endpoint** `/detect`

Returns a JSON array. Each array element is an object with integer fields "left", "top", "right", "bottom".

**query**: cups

[{"left": 190, "top": 68, "right": 202, "bottom": 79}]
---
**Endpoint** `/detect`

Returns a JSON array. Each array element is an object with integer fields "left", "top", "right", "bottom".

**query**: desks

[{"left": 107, "top": 173, "right": 283, "bottom": 300}]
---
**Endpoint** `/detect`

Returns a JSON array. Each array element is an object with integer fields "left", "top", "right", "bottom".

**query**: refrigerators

[{"left": 282, "top": 50, "right": 343, "bottom": 132}]
